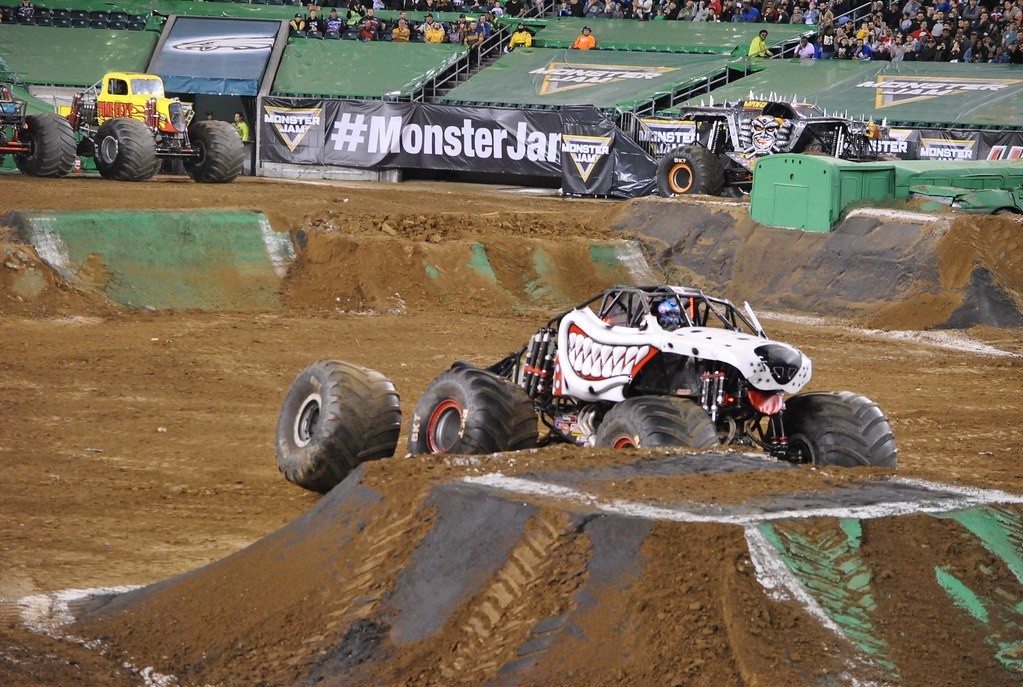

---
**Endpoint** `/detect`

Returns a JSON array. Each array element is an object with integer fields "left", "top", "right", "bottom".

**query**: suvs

[
  {"left": 11, "top": 71, "right": 245, "bottom": 184},
  {"left": 275, "top": 283, "right": 898, "bottom": 494},
  {"left": 657, "top": 89, "right": 887, "bottom": 199}
]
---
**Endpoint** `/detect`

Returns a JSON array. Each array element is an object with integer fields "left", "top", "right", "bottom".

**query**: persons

[
  {"left": 528, "top": 0, "right": 845, "bottom": 26},
  {"left": 813, "top": 0, "right": 1023, "bottom": 63},
  {"left": 793, "top": 37, "right": 814, "bottom": 58},
  {"left": 16, "top": 0, "right": 38, "bottom": 26},
  {"left": 231, "top": 111, "right": 248, "bottom": 142},
  {"left": 657, "top": 300, "right": 681, "bottom": 327},
  {"left": 749, "top": 29, "right": 774, "bottom": 59},
  {"left": 206, "top": 111, "right": 214, "bottom": 120},
  {"left": 572, "top": 26, "right": 596, "bottom": 50},
  {"left": 289, "top": 0, "right": 525, "bottom": 67},
  {"left": 507, "top": 23, "right": 532, "bottom": 52}
]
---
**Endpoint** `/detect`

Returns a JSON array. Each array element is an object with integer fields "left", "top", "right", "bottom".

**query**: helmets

[
  {"left": 658, "top": 301, "right": 680, "bottom": 323},
  {"left": 517, "top": 23, "right": 524, "bottom": 32}
]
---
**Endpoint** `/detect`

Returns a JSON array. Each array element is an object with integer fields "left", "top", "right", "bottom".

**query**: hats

[
  {"left": 295, "top": 13, "right": 301, "bottom": 18},
  {"left": 460, "top": 14, "right": 465, "bottom": 18},
  {"left": 423, "top": 14, "right": 433, "bottom": 18}
]
